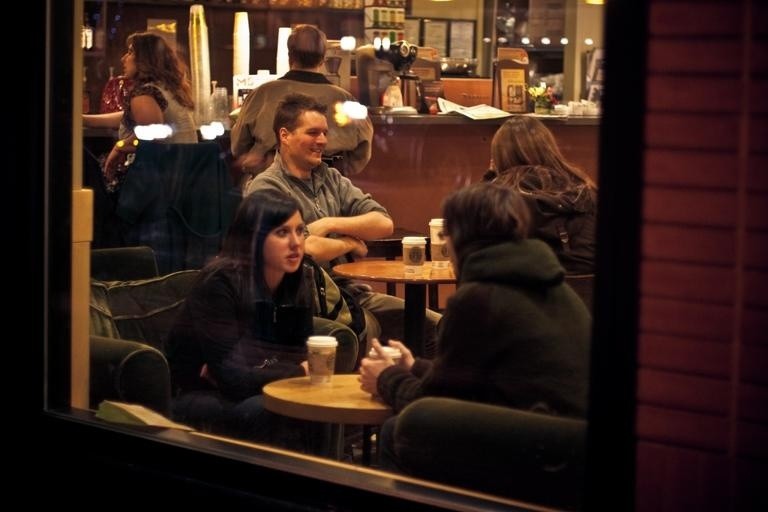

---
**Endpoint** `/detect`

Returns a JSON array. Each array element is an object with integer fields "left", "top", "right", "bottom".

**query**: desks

[
  {"left": 262, "top": 372, "right": 394, "bottom": 463},
  {"left": 333, "top": 260, "right": 458, "bottom": 356}
]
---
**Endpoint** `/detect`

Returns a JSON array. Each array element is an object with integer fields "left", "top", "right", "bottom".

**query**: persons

[
  {"left": 245, "top": 91, "right": 445, "bottom": 353},
  {"left": 356, "top": 182, "right": 587, "bottom": 462},
  {"left": 481, "top": 116, "right": 595, "bottom": 274},
  {"left": 158, "top": 189, "right": 340, "bottom": 448},
  {"left": 229, "top": 24, "right": 376, "bottom": 176},
  {"left": 81, "top": 31, "right": 206, "bottom": 206}
]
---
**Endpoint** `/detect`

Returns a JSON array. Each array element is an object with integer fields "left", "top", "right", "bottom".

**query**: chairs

[
  {"left": 124, "top": 139, "right": 224, "bottom": 275},
  {"left": 390, "top": 396, "right": 594, "bottom": 509},
  {"left": 319, "top": 156, "right": 440, "bottom": 310},
  {"left": 91, "top": 249, "right": 159, "bottom": 286},
  {"left": 91, "top": 277, "right": 201, "bottom": 425}
]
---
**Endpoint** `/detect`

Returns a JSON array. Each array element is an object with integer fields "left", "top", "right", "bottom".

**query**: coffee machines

[{"left": 355, "top": 40, "right": 419, "bottom": 116}]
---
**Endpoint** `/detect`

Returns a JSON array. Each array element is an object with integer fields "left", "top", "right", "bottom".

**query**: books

[{"left": 437, "top": 95, "right": 515, "bottom": 120}]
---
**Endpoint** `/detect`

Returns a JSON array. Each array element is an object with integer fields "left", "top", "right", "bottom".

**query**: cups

[
  {"left": 276, "top": 27, "right": 292, "bottom": 78},
  {"left": 400, "top": 236, "right": 427, "bottom": 275},
  {"left": 306, "top": 335, "right": 338, "bottom": 385},
  {"left": 232, "top": 12, "right": 251, "bottom": 75},
  {"left": 428, "top": 218, "right": 451, "bottom": 269},
  {"left": 187, "top": 5, "right": 212, "bottom": 125},
  {"left": 369, "top": 346, "right": 403, "bottom": 399},
  {"left": 212, "top": 87, "right": 230, "bottom": 122}
]
